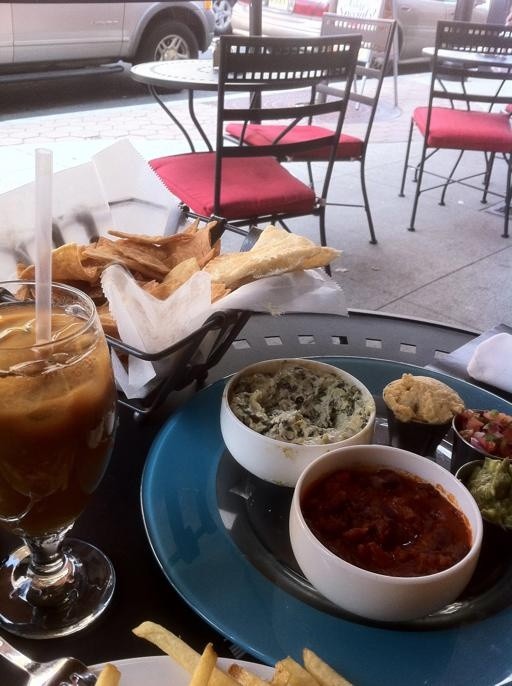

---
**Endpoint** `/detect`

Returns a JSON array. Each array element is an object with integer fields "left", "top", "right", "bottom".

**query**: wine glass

[{"left": 0, "top": 277, "right": 133, "bottom": 645}]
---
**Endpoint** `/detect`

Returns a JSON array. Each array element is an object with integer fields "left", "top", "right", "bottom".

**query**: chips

[{"left": 17, "top": 219, "right": 345, "bottom": 340}]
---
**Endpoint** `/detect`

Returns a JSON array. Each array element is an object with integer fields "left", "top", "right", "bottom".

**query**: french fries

[{"left": 93, "top": 620, "right": 352, "bottom": 686}]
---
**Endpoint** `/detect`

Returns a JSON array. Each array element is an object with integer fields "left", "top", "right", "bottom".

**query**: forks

[{"left": 2, "top": 637, "right": 100, "bottom": 686}]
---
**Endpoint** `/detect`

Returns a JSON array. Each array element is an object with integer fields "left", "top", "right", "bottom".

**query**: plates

[{"left": 63, "top": 654, "right": 299, "bottom": 685}]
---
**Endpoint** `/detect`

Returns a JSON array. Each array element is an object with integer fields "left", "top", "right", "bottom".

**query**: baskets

[{"left": 0, "top": 197, "right": 263, "bottom": 433}]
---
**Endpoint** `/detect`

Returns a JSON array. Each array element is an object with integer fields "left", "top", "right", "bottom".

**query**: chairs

[
  {"left": 217, "top": 12, "right": 400, "bottom": 250},
  {"left": 397, "top": 19, "right": 512, "bottom": 242},
  {"left": 146, "top": 30, "right": 364, "bottom": 280}
]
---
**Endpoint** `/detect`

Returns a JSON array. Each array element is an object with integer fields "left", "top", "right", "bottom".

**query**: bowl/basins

[
  {"left": 287, "top": 437, "right": 484, "bottom": 624},
  {"left": 219, "top": 356, "right": 379, "bottom": 492}
]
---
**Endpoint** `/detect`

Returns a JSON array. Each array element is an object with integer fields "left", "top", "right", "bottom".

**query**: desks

[
  {"left": 397, "top": 40, "right": 512, "bottom": 206},
  {"left": 0, "top": 309, "right": 512, "bottom": 686},
  {"left": 128, "top": 58, "right": 332, "bottom": 153}
]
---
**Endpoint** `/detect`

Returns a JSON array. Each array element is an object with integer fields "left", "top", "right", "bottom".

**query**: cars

[
  {"left": 0, "top": 0, "right": 212, "bottom": 94},
  {"left": 210, "top": 1, "right": 236, "bottom": 34},
  {"left": 230, "top": 0, "right": 497, "bottom": 76}
]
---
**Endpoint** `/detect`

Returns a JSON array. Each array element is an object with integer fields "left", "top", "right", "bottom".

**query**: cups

[
  {"left": 451, "top": 457, "right": 508, "bottom": 565},
  {"left": 378, "top": 370, "right": 469, "bottom": 457},
  {"left": 452, "top": 406, "right": 512, "bottom": 466}
]
